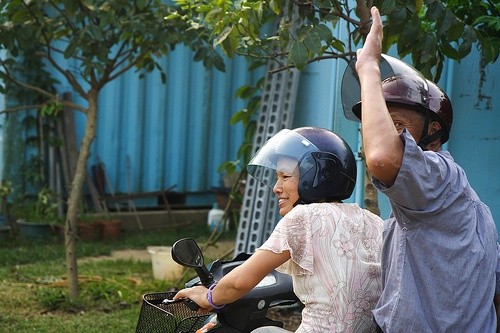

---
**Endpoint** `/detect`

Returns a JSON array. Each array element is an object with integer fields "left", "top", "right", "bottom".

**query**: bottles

[{"left": 207, "top": 203, "right": 229, "bottom": 233}]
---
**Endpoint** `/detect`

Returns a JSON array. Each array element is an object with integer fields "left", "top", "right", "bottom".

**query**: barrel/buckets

[{"left": 147, "top": 246, "right": 184, "bottom": 284}]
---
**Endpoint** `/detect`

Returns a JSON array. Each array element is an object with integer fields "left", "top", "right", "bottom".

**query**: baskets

[{"left": 135, "top": 290, "right": 213, "bottom": 332}]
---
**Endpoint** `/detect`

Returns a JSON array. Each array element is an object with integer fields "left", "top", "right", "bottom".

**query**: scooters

[{"left": 134, "top": 236, "right": 303, "bottom": 333}]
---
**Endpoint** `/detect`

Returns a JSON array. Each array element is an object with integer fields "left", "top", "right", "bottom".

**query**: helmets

[
  {"left": 268, "top": 127, "right": 357, "bottom": 204},
  {"left": 352, "top": 73, "right": 454, "bottom": 144}
]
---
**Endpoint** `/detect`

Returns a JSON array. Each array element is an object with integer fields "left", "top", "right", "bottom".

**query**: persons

[
  {"left": 171, "top": 126, "right": 389, "bottom": 333},
  {"left": 351, "top": 5, "right": 500, "bottom": 333}
]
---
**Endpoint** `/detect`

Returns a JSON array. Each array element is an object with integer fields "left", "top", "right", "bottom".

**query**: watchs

[{"left": 206, "top": 284, "right": 227, "bottom": 309}]
